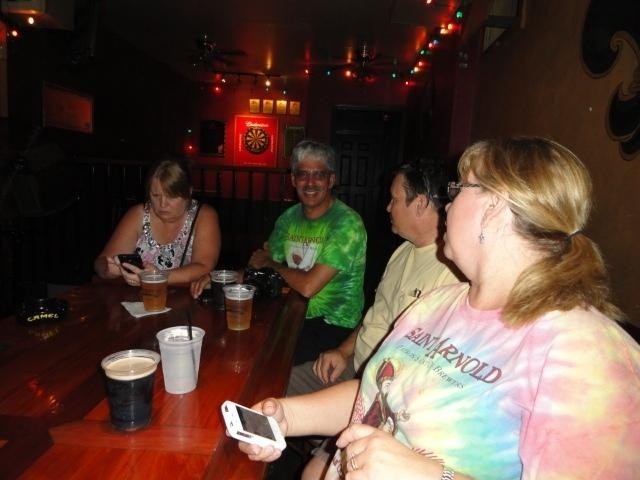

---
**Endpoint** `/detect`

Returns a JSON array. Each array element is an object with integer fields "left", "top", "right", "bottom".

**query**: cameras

[{"left": 241, "top": 264, "right": 285, "bottom": 300}]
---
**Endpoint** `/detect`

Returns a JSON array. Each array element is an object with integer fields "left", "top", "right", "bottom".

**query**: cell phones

[
  {"left": 118, "top": 253, "right": 144, "bottom": 273},
  {"left": 197, "top": 290, "right": 212, "bottom": 303},
  {"left": 220, "top": 398, "right": 288, "bottom": 453}
]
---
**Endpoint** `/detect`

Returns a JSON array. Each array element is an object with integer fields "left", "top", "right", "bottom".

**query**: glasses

[{"left": 448, "top": 181, "right": 480, "bottom": 200}]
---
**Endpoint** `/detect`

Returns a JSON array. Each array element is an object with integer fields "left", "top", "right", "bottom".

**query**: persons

[
  {"left": 93, "top": 155, "right": 221, "bottom": 286},
  {"left": 225, "top": 135, "right": 639, "bottom": 480},
  {"left": 189, "top": 141, "right": 369, "bottom": 362},
  {"left": 282, "top": 153, "right": 467, "bottom": 400}
]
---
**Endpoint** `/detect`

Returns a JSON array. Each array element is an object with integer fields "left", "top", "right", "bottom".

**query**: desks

[{"left": 0, "top": 278, "right": 310, "bottom": 480}]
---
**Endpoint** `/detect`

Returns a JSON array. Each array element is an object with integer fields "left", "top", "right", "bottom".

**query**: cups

[
  {"left": 222, "top": 283, "right": 256, "bottom": 330},
  {"left": 210, "top": 270, "right": 237, "bottom": 310},
  {"left": 101, "top": 349, "right": 161, "bottom": 432},
  {"left": 140, "top": 270, "right": 169, "bottom": 311},
  {"left": 155, "top": 325, "right": 206, "bottom": 394}
]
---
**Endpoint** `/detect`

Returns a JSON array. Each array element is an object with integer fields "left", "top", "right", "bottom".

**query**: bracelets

[{"left": 441, "top": 464, "right": 455, "bottom": 480}]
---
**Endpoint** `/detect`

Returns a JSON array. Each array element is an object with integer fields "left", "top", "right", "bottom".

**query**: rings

[
  {"left": 350, "top": 457, "right": 359, "bottom": 472},
  {"left": 131, "top": 279, "right": 134, "bottom": 285}
]
